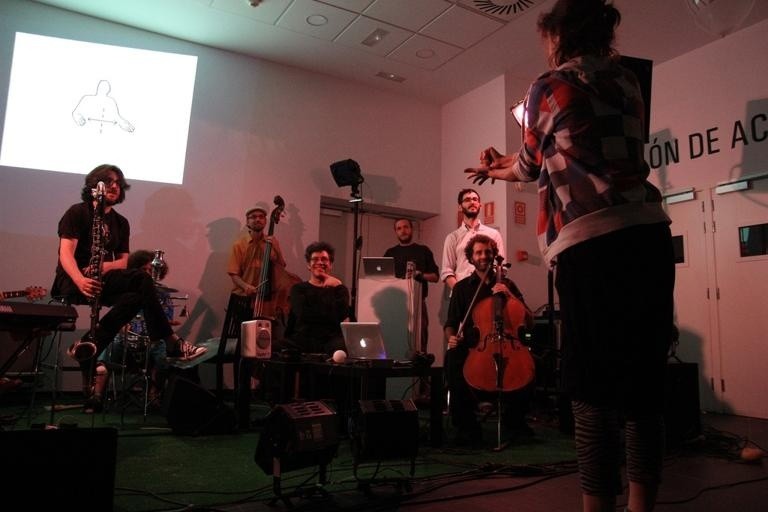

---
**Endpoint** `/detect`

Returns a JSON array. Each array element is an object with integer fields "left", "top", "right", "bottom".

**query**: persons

[
  {"left": 50, "top": 164, "right": 208, "bottom": 362},
  {"left": 442, "top": 234, "right": 535, "bottom": 450},
  {"left": 83, "top": 249, "right": 175, "bottom": 413},
  {"left": 227, "top": 206, "right": 288, "bottom": 299},
  {"left": 382, "top": 217, "right": 441, "bottom": 397},
  {"left": 283, "top": 242, "right": 358, "bottom": 355},
  {"left": 464, "top": 0, "right": 676, "bottom": 512},
  {"left": 441, "top": 189, "right": 508, "bottom": 414}
]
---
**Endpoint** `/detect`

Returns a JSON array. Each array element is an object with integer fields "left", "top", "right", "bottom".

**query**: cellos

[
  {"left": 463, "top": 255, "right": 535, "bottom": 391},
  {"left": 251, "top": 196, "right": 303, "bottom": 338}
]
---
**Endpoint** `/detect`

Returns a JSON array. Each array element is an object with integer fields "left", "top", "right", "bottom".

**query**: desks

[{"left": 263, "top": 350, "right": 445, "bottom": 452}]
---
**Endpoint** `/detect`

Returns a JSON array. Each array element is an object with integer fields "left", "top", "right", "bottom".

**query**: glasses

[
  {"left": 248, "top": 215, "right": 264, "bottom": 219},
  {"left": 462, "top": 197, "right": 478, "bottom": 202},
  {"left": 104, "top": 178, "right": 125, "bottom": 188}
]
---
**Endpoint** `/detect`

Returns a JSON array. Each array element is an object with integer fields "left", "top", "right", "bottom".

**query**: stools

[{"left": 26, "top": 296, "right": 97, "bottom": 425}]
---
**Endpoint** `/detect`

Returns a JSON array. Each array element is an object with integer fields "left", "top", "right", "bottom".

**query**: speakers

[
  {"left": 329, "top": 159, "right": 361, "bottom": 188},
  {"left": 240, "top": 320, "right": 272, "bottom": 358}
]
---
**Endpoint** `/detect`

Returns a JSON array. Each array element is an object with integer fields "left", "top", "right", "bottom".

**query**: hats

[{"left": 245, "top": 207, "right": 267, "bottom": 215}]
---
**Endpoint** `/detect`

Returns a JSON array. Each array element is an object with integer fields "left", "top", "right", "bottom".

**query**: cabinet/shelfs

[{"left": 357, "top": 278, "right": 423, "bottom": 403}]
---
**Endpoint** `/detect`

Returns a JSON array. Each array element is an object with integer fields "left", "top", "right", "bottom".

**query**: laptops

[
  {"left": 340, "top": 320, "right": 386, "bottom": 360},
  {"left": 362, "top": 256, "right": 395, "bottom": 278}
]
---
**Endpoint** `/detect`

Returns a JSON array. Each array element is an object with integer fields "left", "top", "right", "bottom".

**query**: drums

[{"left": 112, "top": 336, "right": 150, "bottom": 372}]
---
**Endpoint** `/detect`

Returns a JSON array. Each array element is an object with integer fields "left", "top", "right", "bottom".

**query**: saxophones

[{"left": 71, "top": 180, "right": 109, "bottom": 399}]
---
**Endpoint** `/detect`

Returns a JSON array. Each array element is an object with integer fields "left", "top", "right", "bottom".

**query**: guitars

[{"left": 0, "top": 285, "right": 48, "bottom": 304}]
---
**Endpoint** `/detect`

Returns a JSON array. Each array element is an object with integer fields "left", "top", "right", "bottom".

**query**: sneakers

[
  {"left": 67, "top": 339, "right": 96, "bottom": 363},
  {"left": 165, "top": 336, "right": 208, "bottom": 361}
]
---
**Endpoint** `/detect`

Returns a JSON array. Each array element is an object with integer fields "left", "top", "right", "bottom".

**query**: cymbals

[{"left": 155, "top": 283, "right": 178, "bottom": 294}]
